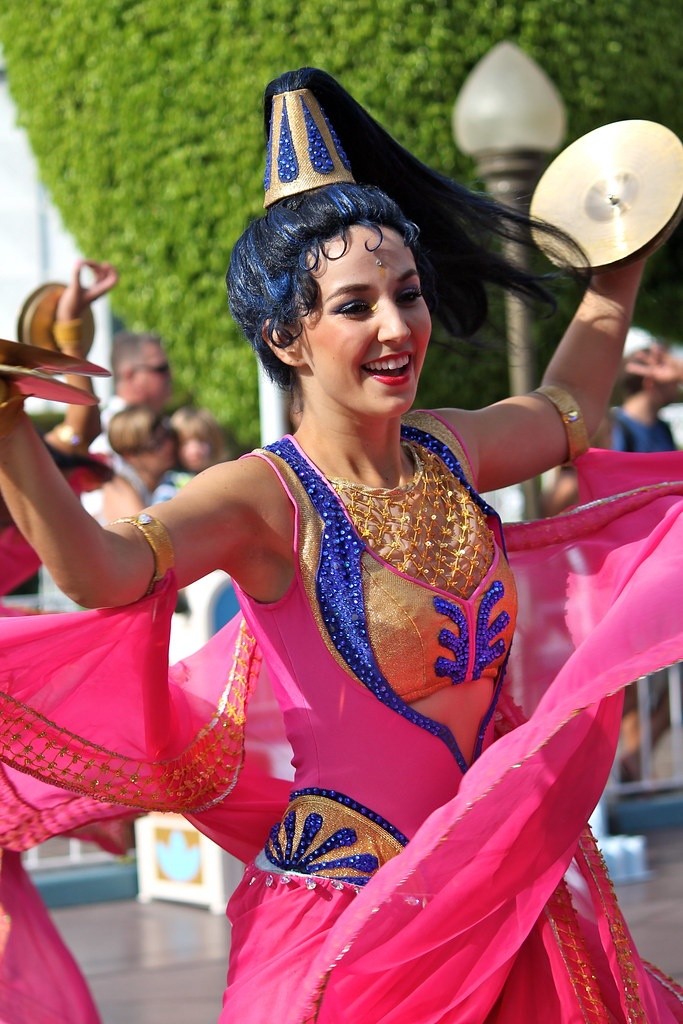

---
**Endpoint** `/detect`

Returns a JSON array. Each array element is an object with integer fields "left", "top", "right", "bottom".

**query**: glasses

[{"left": 135, "top": 362, "right": 169, "bottom": 375}]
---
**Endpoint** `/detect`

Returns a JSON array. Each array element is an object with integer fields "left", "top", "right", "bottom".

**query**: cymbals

[
  {"left": 527, "top": 118, "right": 683, "bottom": 272},
  {"left": 0, "top": 337, "right": 114, "bottom": 408},
  {"left": 15, "top": 281, "right": 97, "bottom": 364}
]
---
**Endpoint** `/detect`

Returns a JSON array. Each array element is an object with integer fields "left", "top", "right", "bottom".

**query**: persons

[
  {"left": 86, "top": 333, "right": 180, "bottom": 503},
  {"left": 1, "top": 259, "right": 119, "bottom": 1024},
  {"left": 608, "top": 325, "right": 682, "bottom": 785},
  {"left": 151, "top": 408, "right": 230, "bottom": 509},
  {"left": 1, "top": 70, "right": 683, "bottom": 1024},
  {"left": 37, "top": 401, "right": 178, "bottom": 613}
]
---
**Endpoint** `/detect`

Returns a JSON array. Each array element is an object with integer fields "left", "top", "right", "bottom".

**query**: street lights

[{"left": 452, "top": 40, "right": 567, "bottom": 524}]
